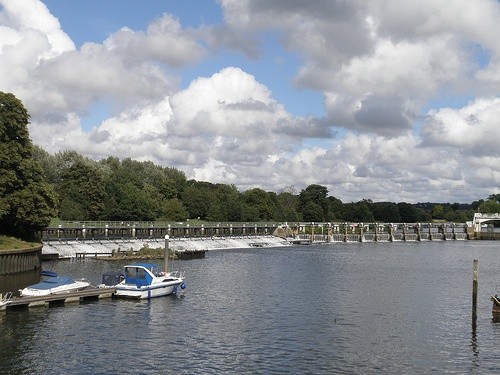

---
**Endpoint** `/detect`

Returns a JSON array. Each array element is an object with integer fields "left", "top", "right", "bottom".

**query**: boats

[
  {"left": 97, "top": 263, "right": 186, "bottom": 300},
  {"left": 19, "top": 271, "right": 90, "bottom": 297}
]
